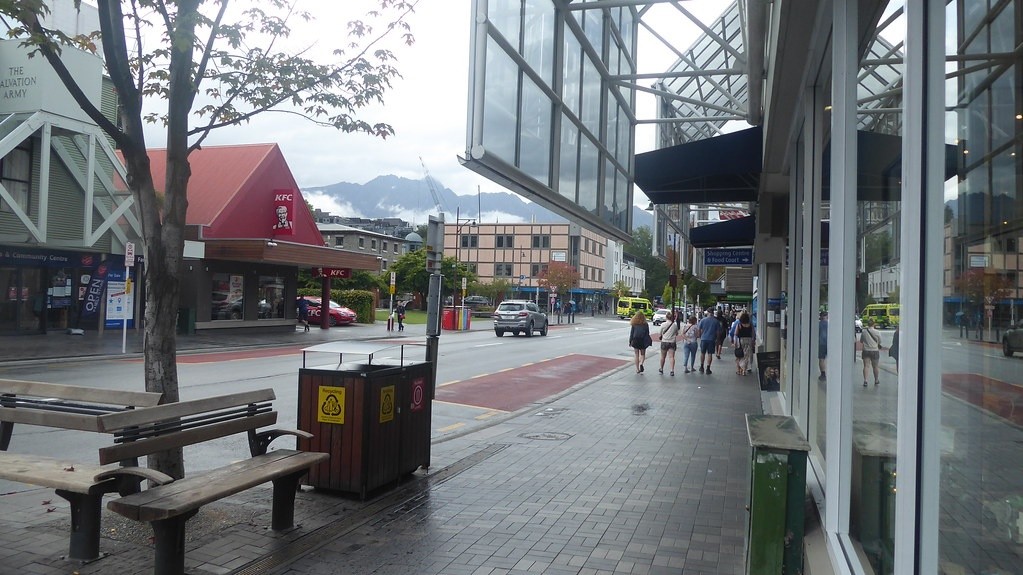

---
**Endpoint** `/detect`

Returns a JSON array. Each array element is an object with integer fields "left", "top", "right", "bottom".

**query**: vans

[
  {"left": 617, "top": 297, "right": 656, "bottom": 320},
  {"left": 862, "top": 304, "right": 901, "bottom": 329}
]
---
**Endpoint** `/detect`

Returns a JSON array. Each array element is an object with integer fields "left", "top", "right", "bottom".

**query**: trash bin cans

[
  {"left": 443, "top": 306, "right": 471, "bottom": 330},
  {"left": 296, "top": 341, "right": 433, "bottom": 499}
]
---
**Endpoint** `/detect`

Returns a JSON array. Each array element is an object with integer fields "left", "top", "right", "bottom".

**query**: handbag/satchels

[
  {"left": 734, "top": 348, "right": 744, "bottom": 358},
  {"left": 659, "top": 336, "right": 662, "bottom": 340},
  {"left": 631, "top": 338, "right": 643, "bottom": 349},
  {"left": 878, "top": 345, "right": 881, "bottom": 349}
]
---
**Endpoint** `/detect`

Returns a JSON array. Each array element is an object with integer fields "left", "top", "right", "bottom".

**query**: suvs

[{"left": 493, "top": 300, "right": 549, "bottom": 338}]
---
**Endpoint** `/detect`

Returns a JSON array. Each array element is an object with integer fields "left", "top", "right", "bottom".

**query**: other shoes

[
  {"left": 743, "top": 372, "right": 746, "bottom": 376},
  {"left": 717, "top": 356, "right": 721, "bottom": 359},
  {"left": 747, "top": 369, "right": 751, "bottom": 372},
  {"left": 864, "top": 382, "right": 868, "bottom": 386},
  {"left": 699, "top": 368, "right": 704, "bottom": 372},
  {"left": 706, "top": 370, "right": 712, "bottom": 374},
  {"left": 819, "top": 376, "right": 826, "bottom": 380},
  {"left": 875, "top": 381, "right": 879, "bottom": 384},
  {"left": 736, "top": 371, "right": 740, "bottom": 375},
  {"left": 670, "top": 372, "right": 674, "bottom": 376},
  {"left": 659, "top": 368, "right": 663, "bottom": 375},
  {"left": 684, "top": 370, "right": 690, "bottom": 373},
  {"left": 691, "top": 368, "right": 696, "bottom": 371},
  {"left": 640, "top": 365, "right": 644, "bottom": 375}
]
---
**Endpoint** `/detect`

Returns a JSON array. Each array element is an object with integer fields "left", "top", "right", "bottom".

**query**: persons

[
  {"left": 674, "top": 305, "right": 747, "bottom": 335},
  {"left": 697, "top": 307, "right": 720, "bottom": 374},
  {"left": 734, "top": 313, "right": 756, "bottom": 376},
  {"left": 889, "top": 326, "right": 898, "bottom": 372},
  {"left": 297, "top": 294, "right": 310, "bottom": 331},
  {"left": 859, "top": 318, "right": 883, "bottom": 387},
  {"left": 628, "top": 311, "right": 652, "bottom": 373},
  {"left": 681, "top": 317, "right": 700, "bottom": 373},
  {"left": 818, "top": 311, "right": 828, "bottom": 380},
  {"left": 714, "top": 311, "right": 728, "bottom": 357},
  {"left": 393, "top": 301, "right": 405, "bottom": 331},
  {"left": 658, "top": 312, "right": 679, "bottom": 376},
  {"left": 555, "top": 300, "right": 610, "bottom": 315},
  {"left": 854, "top": 322, "right": 857, "bottom": 362},
  {"left": 730, "top": 311, "right": 745, "bottom": 375}
]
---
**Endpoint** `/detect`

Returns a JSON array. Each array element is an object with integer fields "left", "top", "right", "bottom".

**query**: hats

[
  {"left": 707, "top": 307, "right": 714, "bottom": 313},
  {"left": 717, "top": 311, "right": 723, "bottom": 315},
  {"left": 820, "top": 311, "right": 828, "bottom": 316}
]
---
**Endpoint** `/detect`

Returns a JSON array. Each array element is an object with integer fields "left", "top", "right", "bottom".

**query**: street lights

[{"left": 452, "top": 206, "right": 477, "bottom": 328}]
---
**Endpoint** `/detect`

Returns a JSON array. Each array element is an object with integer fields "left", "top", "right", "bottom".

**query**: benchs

[
  {"left": 98, "top": 388, "right": 330, "bottom": 575},
  {"left": 0, "top": 379, "right": 164, "bottom": 564}
]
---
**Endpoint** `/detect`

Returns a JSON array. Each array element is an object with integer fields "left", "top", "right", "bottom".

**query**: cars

[
  {"left": 295, "top": 296, "right": 357, "bottom": 327},
  {"left": 675, "top": 306, "right": 702, "bottom": 320},
  {"left": 653, "top": 309, "right": 674, "bottom": 324},
  {"left": 854, "top": 315, "right": 863, "bottom": 332},
  {"left": 465, "top": 294, "right": 490, "bottom": 306},
  {"left": 1000, "top": 318, "right": 1023, "bottom": 357}
]
